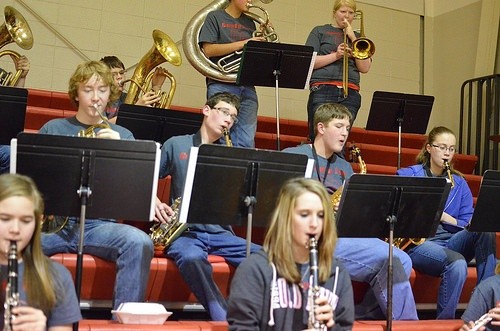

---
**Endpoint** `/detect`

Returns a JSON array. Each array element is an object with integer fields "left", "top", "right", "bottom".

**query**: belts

[{"left": 321, "top": 83, "right": 343, "bottom": 89}]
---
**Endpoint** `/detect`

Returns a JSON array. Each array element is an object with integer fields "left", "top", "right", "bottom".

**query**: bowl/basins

[{"left": 111, "top": 302, "right": 173, "bottom": 324}]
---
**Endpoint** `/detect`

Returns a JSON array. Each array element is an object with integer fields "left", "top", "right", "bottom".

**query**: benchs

[{"left": 23, "top": 88, "right": 500, "bottom": 330}]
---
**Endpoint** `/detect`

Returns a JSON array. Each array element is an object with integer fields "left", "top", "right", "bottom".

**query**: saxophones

[
  {"left": 149, "top": 126, "right": 233, "bottom": 247},
  {"left": 466, "top": 307, "right": 500, "bottom": 331},
  {"left": 388, "top": 159, "right": 455, "bottom": 251},
  {"left": 332, "top": 143, "right": 366, "bottom": 221},
  {"left": 40, "top": 103, "right": 112, "bottom": 235}
]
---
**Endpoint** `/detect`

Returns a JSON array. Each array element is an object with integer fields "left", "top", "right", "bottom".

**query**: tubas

[
  {"left": 0, "top": 6, "right": 34, "bottom": 87},
  {"left": 115, "top": 30, "right": 182, "bottom": 109}
]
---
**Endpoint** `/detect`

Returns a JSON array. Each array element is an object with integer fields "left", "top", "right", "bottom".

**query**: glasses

[
  {"left": 213, "top": 107, "right": 238, "bottom": 124},
  {"left": 432, "top": 145, "right": 456, "bottom": 152},
  {"left": 112, "top": 70, "right": 127, "bottom": 77}
]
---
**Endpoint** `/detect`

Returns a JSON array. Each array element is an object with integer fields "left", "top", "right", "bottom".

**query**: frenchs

[{"left": 182, "top": 0, "right": 279, "bottom": 83}]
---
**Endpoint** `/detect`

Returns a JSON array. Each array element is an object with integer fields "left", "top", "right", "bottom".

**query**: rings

[{"left": 160, "top": 209, "right": 164, "bottom": 211}]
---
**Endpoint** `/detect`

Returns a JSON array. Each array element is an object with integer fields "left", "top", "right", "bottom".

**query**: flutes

[
  {"left": 2, "top": 240, "right": 20, "bottom": 331},
  {"left": 304, "top": 233, "right": 328, "bottom": 330}
]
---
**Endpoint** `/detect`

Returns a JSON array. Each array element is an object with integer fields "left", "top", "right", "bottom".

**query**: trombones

[{"left": 340, "top": 9, "right": 376, "bottom": 98}]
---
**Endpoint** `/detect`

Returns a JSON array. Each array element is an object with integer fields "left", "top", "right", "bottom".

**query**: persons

[
  {"left": 305, "top": 0, "right": 372, "bottom": 142},
  {"left": 198, "top": 0, "right": 274, "bottom": 148},
  {"left": 394, "top": 127, "right": 496, "bottom": 320},
  {"left": 40, "top": 60, "right": 154, "bottom": 318},
  {"left": 0, "top": 54, "right": 30, "bottom": 174},
  {"left": 0, "top": 174, "right": 82, "bottom": 331},
  {"left": 280, "top": 104, "right": 419, "bottom": 320},
  {"left": 153, "top": 92, "right": 263, "bottom": 320},
  {"left": 226, "top": 179, "right": 354, "bottom": 331},
  {"left": 100, "top": 56, "right": 168, "bottom": 107},
  {"left": 460, "top": 275, "right": 500, "bottom": 331}
]
